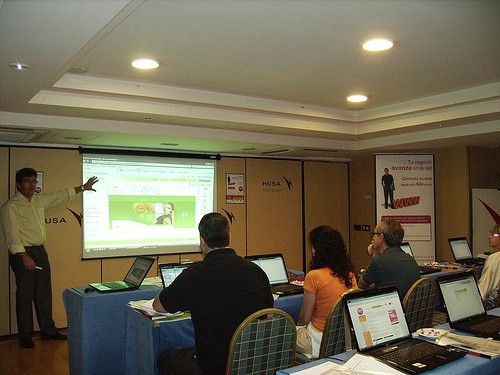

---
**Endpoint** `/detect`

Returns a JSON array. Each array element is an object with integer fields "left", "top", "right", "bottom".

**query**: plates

[
  {"left": 417, "top": 328, "right": 449, "bottom": 339},
  {"left": 424, "top": 262, "right": 438, "bottom": 266},
  {"left": 290, "top": 280, "right": 305, "bottom": 286}
]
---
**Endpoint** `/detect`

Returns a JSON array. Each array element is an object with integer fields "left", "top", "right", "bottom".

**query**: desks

[
  {"left": 62, "top": 268, "right": 305, "bottom": 375},
  {"left": 277, "top": 306, "right": 500, "bottom": 375},
  {"left": 124, "top": 258, "right": 481, "bottom": 375}
]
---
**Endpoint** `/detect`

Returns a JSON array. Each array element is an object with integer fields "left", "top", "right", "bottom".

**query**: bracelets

[{"left": 80, "top": 185, "right": 84, "bottom": 191}]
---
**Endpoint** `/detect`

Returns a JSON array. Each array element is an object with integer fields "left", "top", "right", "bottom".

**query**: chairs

[
  {"left": 403, "top": 277, "right": 436, "bottom": 333},
  {"left": 296, "top": 288, "right": 365, "bottom": 366},
  {"left": 227, "top": 307, "right": 297, "bottom": 375}
]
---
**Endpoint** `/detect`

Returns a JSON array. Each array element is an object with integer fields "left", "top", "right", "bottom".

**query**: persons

[
  {"left": 1, "top": 168, "right": 99, "bottom": 348},
  {"left": 357, "top": 219, "right": 424, "bottom": 345},
  {"left": 153, "top": 213, "right": 275, "bottom": 375},
  {"left": 381, "top": 168, "right": 397, "bottom": 209},
  {"left": 477, "top": 226, "right": 500, "bottom": 310},
  {"left": 295, "top": 225, "right": 359, "bottom": 360}
]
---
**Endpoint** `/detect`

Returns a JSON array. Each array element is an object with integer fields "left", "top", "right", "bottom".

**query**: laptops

[
  {"left": 400, "top": 242, "right": 442, "bottom": 274},
  {"left": 342, "top": 286, "right": 468, "bottom": 375},
  {"left": 438, "top": 274, "right": 500, "bottom": 342},
  {"left": 159, "top": 263, "right": 191, "bottom": 289},
  {"left": 89, "top": 256, "right": 155, "bottom": 293},
  {"left": 448, "top": 237, "right": 486, "bottom": 265},
  {"left": 244, "top": 253, "right": 304, "bottom": 296}
]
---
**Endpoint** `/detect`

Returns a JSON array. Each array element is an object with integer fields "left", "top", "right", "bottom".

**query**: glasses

[
  {"left": 367, "top": 230, "right": 384, "bottom": 238},
  {"left": 23, "top": 178, "right": 39, "bottom": 184}
]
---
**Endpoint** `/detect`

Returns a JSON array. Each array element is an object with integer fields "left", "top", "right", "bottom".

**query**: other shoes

[
  {"left": 19, "top": 336, "right": 35, "bottom": 348},
  {"left": 41, "top": 331, "right": 68, "bottom": 341}
]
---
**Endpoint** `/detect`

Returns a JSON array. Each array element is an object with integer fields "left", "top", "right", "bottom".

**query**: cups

[{"left": 290, "top": 274, "right": 303, "bottom": 281}]
